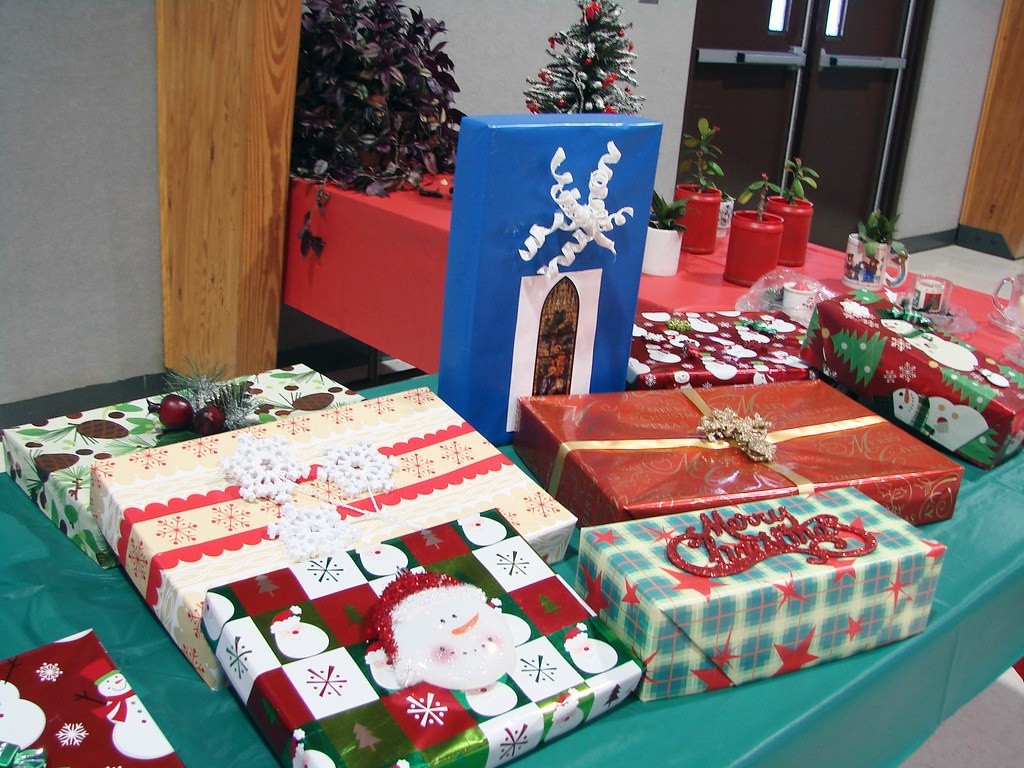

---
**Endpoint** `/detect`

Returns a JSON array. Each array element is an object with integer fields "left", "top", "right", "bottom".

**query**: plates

[
  {"left": 1002, "top": 343, "right": 1024, "bottom": 367},
  {"left": 988, "top": 312, "right": 1024, "bottom": 337}
]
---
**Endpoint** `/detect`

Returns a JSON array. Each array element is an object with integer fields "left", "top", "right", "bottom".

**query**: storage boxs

[
  {"left": 89, "top": 388, "right": 579, "bottom": 692},
  {"left": 802, "top": 288, "right": 1024, "bottom": 470},
  {"left": 0, "top": 363, "right": 368, "bottom": 570},
  {"left": 437, "top": 115, "right": 663, "bottom": 448},
  {"left": 517, "top": 381, "right": 967, "bottom": 527},
  {"left": 624, "top": 311, "right": 813, "bottom": 390},
  {"left": 200, "top": 509, "right": 647, "bottom": 768},
  {"left": 0, "top": 628, "right": 188, "bottom": 768},
  {"left": 575, "top": 486, "right": 948, "bottom": 705}
]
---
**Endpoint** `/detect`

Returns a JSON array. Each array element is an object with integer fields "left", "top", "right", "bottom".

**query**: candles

[{"left": 913, "top": 278, "right": 945, "bottom": 313}]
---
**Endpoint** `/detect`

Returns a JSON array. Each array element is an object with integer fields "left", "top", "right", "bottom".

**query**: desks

[
  {"left": 282, "top": 173, "right": 1024, "bottom": 375},
  {"left": 0, "top": 365, "right": 1024, "bottom": 768}
]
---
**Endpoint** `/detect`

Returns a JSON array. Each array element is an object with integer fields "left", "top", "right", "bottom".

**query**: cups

[
  {"left": 992, "top": 272, "right": 1024, "bottom": 328},
  {"left": 912, "top": 274, "right": 954, "bottom": 316},
  {"left": 783, "top": 282, "right": 817, "bottom": 329}
]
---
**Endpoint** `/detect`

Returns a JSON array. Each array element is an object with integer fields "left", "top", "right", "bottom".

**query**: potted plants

[
  {"left": 842, "top": 209, "right": 909, "bottom": 291},
  {"left": 766, "top": 158, "right": 819, "bottom": 267},
  {"left": 722, "top": 172, "right": 785, "bottom": 289},
  {"left": 641, "top": 189, "right": 691, "bottom": 278},
  {"left": 674, "top": 119, "right": 724, "bottom": 254}
]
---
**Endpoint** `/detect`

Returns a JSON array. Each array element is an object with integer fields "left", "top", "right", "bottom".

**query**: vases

[{"left": 781, "top": 282, "right": 817, "bottom": 321}]
